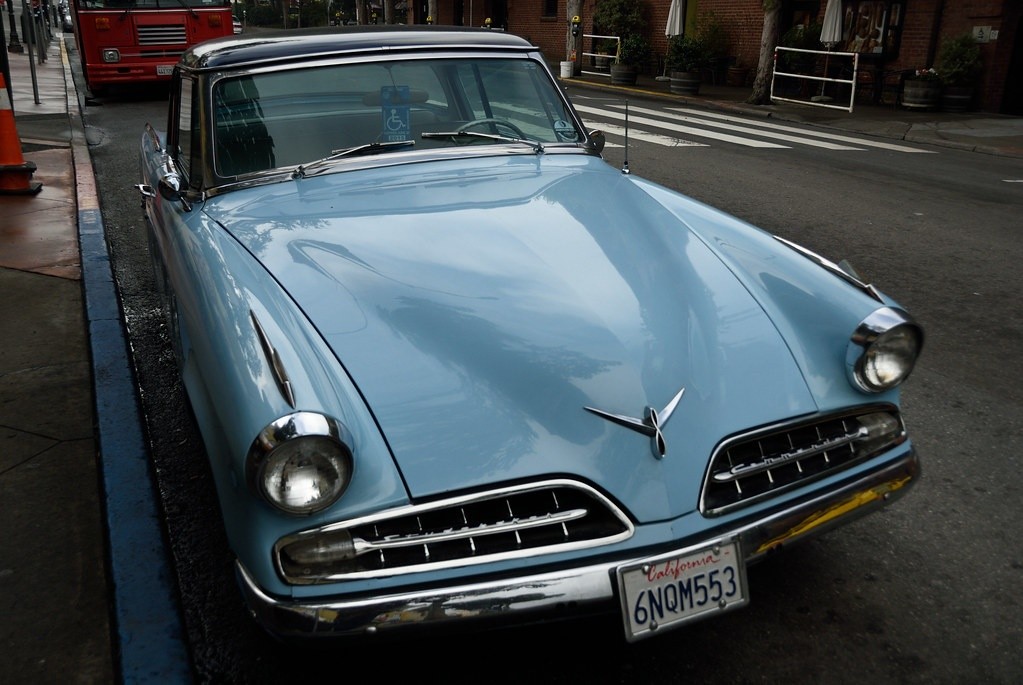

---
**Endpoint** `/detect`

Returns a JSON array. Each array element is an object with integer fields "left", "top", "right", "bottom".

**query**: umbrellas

[
  {"left": 664, "top": 0, "right": 683, "bottom": 79},
  {"left": 820, "top": 0, "right": 843, "bottom": 95}
]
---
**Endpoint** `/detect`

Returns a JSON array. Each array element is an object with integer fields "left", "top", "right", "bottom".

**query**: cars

[
  {"left": 132, "top": 24, "right": 929, "bottom": 673},
  {"left": 232, "top": 14, "right": 243, "bottom": 34},
  {"left": 58, "top": 0, "right": 74, "bottom": 31}
]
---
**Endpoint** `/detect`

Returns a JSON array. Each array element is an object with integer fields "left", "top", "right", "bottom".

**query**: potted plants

[
  {"left": 941, "top": 30, "right": 985, "bottom": 111},
  {"left": 595, "top": 40, "right": 612, "bottom": 67},
  {"left": 611, "top": 34, "right": 654, "bottom": 87},
  {"left": 724, "top": 53, "right": 747, "bottom": 87},
  {"left": 664, "top": 33, "right": 708, "bottom": 95}
]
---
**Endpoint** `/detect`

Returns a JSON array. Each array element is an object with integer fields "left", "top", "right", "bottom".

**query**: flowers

[{"left": 910, "top": 67, "right": 944, "bottom": 82}]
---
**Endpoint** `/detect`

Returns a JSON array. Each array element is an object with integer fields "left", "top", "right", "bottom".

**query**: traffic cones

[{"left": 0, "top": 70, "right": 44, "bottom": 196}]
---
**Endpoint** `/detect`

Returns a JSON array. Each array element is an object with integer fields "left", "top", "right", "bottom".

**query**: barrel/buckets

[{"left": 560, "top": 61, "right": 574, "bottom": 79}]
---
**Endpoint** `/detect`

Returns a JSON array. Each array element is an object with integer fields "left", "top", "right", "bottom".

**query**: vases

[{"left": 903, "top": 79, "right": 940, "bottom": 108}]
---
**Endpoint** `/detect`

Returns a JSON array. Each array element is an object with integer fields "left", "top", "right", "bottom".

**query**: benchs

[{"left": 214, "top": 107, "right": 438, "bottom": 178}]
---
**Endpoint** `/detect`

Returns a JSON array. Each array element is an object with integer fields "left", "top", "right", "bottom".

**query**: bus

[{"left": 67, "top": 0, "right": 236, "bottom": 101}]
